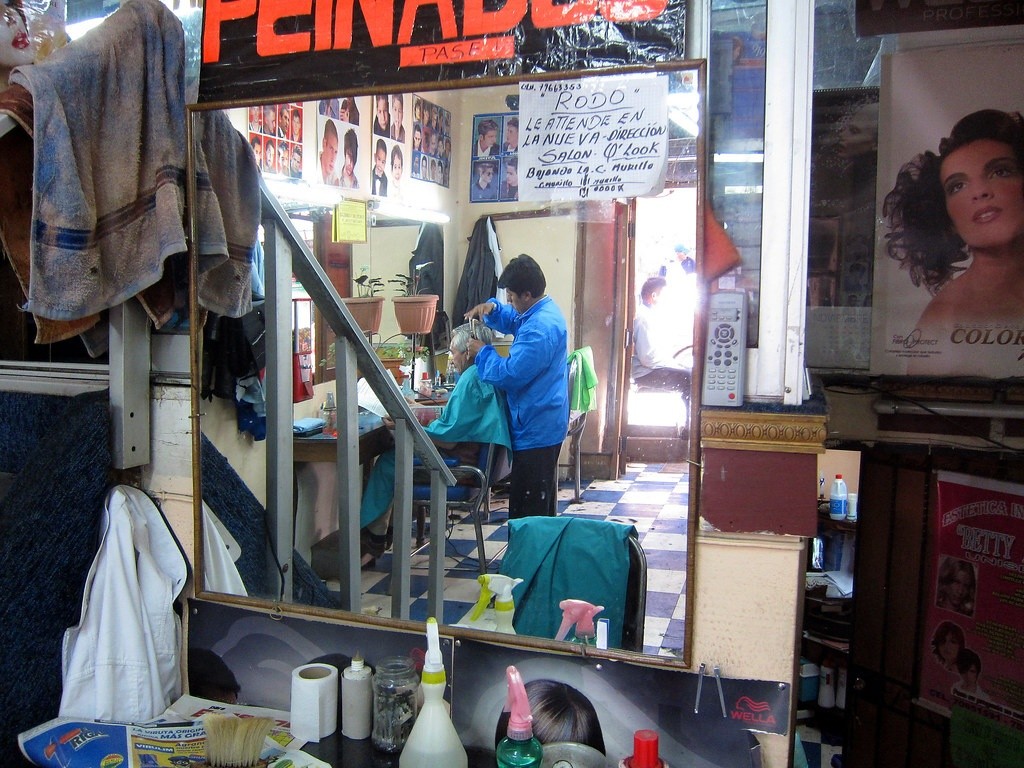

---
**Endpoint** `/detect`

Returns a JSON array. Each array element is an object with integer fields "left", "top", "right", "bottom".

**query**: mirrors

[
  {"left": 183, "top": 59, "right": 707, "bottom": 668},
  {"left": 290, "top": 204, "right": 443, "bottom": 383}
]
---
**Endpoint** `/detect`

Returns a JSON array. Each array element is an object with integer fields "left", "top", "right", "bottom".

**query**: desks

[
  {"left": 243, "top": 702, "right": 498, "bottom": 768},
  {"left": 293, "top": 408, "right": 394, "bottom": 547}
]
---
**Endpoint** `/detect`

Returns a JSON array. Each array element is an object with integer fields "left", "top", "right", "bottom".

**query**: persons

[
  {"left": 471, "top": 164, "right": 497, "bottom": 200},
  {"left": 464, "top": 254, "right": 568, "bottom": 543},
  {"left": 374, "top": 94, "right": 390, "bottom": 138},
  {"left": 318, "top": 98, "right": 339, "bottom": 120},
  {"left": 248, "top": 101, "right": 302, "bottom": 179},
  {"left": 472, "top": 120, "right": 499, "bottom": 155},
  {"left": 501, "top": 116, "right": 518, "bottom": 199},
  {"left": 881, "top": 106, "right": 1024, "bottom": 376},
  {"left": 337, "top": 128, "right": 361, "bottom": 189},
  {"left": 930, "top": 557, "right": 991, "bottom": 702},
  {"left": 412, "top": 97, "right": 452, "bottom": 187},
  {"left": 360, "top": 321, "right": 492, "bottom": 561},
  {"left": 339, "top": 96, "right": 359, "bottom": 126},
  {"left": 372, "top": 139, "right": 388, "bottom": 197},
  {"left": 390, "top": 93, "right": 405, "bottom": 143},
  {"left": 318, "top": 119, "right": 340, "bottom": 186},
  {"left": 388, "top": 144, "right": 404, "bottom": 197},
  {"left": 628, "top": 278, "right": 689, "bottom": 425},
  {"left": 674, "top": 245, "right": 696, "bottom": 274},
  {"left": 0, "top": 0, "right": 37, "bottom": 93}
]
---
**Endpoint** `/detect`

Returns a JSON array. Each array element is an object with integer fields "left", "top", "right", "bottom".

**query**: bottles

[
  {"left": 323, "top": 393, "right": 337, "bottom": 433},
  {"left": 446, "top": 356, "right": 454, "bottom": 383},
  {"left": 370, "top": 654, "right": 420, "bottom": 750},
  {"left": 343, "top": 649, "right": 372, "bottom": 680},
  {"left": 419, "top": 372, "right": 432, "bottom": 396},
  {"left": 402, "top": 374, "right": 415, "bottom": 402},
  {"left": 829, "top": 474, "right": 857, "bottom": 520},
  {"left": 435, "top": 369, "right": 441, "bottom": 386}
]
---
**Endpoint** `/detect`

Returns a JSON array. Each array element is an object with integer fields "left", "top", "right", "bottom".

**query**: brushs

[{"left": 189, "top": 714, "right": 276, "bottom": 768}]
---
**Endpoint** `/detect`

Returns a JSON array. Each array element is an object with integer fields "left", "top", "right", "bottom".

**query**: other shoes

[
  {"left": 384, "top": 533, "right": 393, "bottom": 549},
  {"left": 359, "top": 528, "right": 390, "bottom": 555}
]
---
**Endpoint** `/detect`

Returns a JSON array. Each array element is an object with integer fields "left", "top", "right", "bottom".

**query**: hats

[{"left": 673, "top": 245, "right": 687, "bottom": 254}]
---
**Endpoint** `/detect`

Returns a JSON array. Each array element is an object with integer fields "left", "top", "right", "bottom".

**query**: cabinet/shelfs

[{"left": 795, "top": 440, "right": 887, "bottom": 768}]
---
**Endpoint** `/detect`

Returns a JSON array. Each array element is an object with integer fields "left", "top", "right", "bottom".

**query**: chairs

[
  {"left": 413, "top": 442, "right": 495, "bottom": 575},
  {"left": 503, "top": 516, "right": 647, "bottom": 652},
  {"left": 555, "top": 345, "right": 598, "bottom": 516}
]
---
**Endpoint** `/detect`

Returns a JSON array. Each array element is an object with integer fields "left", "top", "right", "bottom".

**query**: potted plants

[
  {"left": 340, "top": 266, "right": 384, "bottom": 335},
  {"left": 359, "top": 345, "right": 405, "bottom": 386},
  {"left": 387, "top": 247, "right": 440, "bottom": 334}
]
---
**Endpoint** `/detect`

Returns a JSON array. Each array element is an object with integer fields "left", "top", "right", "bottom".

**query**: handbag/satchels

[{"left": 222, "top": 302, "right": 265, "bottom": 376}]
